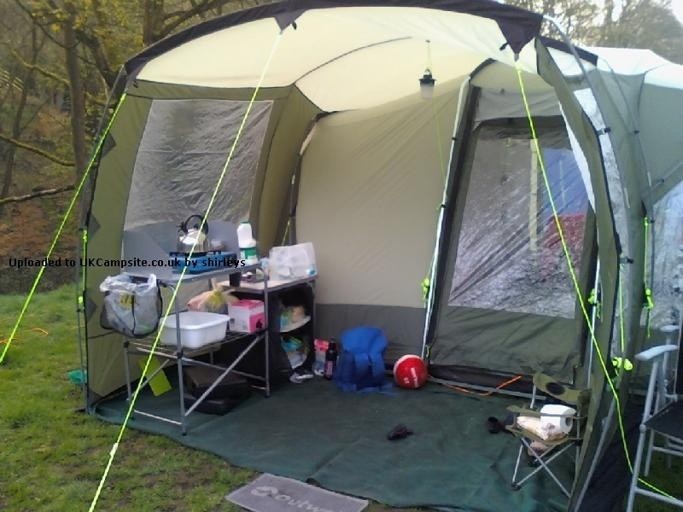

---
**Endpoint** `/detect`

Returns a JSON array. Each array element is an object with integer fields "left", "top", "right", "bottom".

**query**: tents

[{"left": 80, "top": 2, "right": 683, "bottom": 511}]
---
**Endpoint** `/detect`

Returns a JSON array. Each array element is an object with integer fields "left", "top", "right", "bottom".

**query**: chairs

[
  {"left": 642, "top": 325, "right": 682, "bottom": 478},
  {"left": 626, "top": 305, "right": 682, "bottom": 512},
  {"left": 503, "top": 371, "right": 586, "bottom": 500}
]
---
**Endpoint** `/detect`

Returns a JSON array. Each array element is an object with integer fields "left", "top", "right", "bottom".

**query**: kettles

[{"left": 179, "top": 216, "right": 208, "bottom": 256}]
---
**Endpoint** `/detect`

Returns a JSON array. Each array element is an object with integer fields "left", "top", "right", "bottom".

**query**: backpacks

[{"left": 336, "top": 325, "right": 387, "bottom": 391}]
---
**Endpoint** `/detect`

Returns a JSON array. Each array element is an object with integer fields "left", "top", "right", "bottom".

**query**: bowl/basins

[{"left": 157, "top": 309, "right": 231, "bottom": 349}]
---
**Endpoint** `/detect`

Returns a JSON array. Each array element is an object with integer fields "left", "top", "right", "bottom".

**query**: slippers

[{"left": 487, "top": 416, "right": 501, "bottom": 434}]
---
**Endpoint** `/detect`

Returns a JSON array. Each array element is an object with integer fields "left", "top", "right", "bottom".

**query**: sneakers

[{"left": 387, "top": 424, "right": 413, "bottom": 441}]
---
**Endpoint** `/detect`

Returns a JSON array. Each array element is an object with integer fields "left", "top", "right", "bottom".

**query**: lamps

[{"left": 418, "top": 40, "right": 436, "bottom": 100}]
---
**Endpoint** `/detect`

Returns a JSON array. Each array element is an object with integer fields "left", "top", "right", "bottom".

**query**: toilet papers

[{"left": 540, "top": 404, "right": 575, "bottom": 434}]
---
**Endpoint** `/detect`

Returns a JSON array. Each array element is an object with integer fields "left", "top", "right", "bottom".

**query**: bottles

[{"left": 323, "top": 338, "right": 337, "bottom": 381}]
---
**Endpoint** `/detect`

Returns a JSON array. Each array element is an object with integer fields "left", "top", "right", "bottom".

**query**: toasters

[{"left": 167, "top": 251, "right": 237, "bottom": 273}]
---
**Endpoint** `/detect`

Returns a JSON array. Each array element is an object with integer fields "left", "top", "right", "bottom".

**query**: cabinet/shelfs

[
  {"left": 121, "top": 258, "right": 271, "bottom": 437},
  {"left": 215, "top": 276, "right": 320, "bottom": 393}
]
